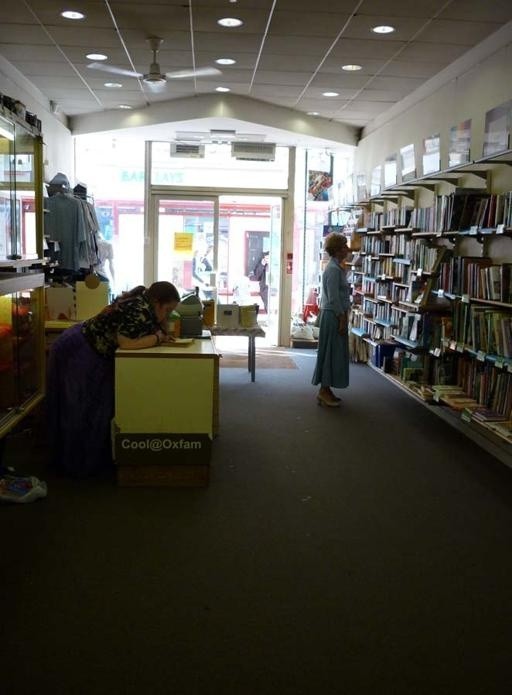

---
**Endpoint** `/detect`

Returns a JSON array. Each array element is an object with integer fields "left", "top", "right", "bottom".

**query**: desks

[{"left": 198, "top": 326, "right": 266, "bottom": 383}]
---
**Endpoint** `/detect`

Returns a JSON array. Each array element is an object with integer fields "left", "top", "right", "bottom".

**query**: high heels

[{"left": 317, "top": 393, "right": 344, "bottom": 407}]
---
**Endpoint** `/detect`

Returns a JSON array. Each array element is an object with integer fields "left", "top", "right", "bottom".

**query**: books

[
  {"left": 163, "top": 338, "right": 194, "bottom": 348},
  {"left": 344, "top": 188, "right": 511, "bottom": 442}
]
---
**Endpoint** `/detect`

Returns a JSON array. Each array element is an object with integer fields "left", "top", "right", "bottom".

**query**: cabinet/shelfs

[{"left": 328, "top": 145, "right": 511, "bottom": 469}]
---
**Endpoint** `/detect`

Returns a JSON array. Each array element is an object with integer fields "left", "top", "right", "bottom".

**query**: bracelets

[{"left": 153, "top": 334, "right": 160, "bottom": 344}]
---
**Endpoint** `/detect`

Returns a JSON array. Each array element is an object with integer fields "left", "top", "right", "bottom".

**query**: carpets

[{"left": 219, "top": 345, "right": 298, "bottom": 369}]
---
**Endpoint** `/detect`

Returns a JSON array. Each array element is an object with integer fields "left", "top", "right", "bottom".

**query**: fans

[{"left": 88, "top": 36, "right": 223, "bottom": 88}]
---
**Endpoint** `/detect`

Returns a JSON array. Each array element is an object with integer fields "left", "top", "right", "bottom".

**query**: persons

[
  {"left": 191, "top": 241, "right": 212, "bottom": 304},
  {"left": 9, "top": 278, "right": 181, "bottom": 493},
  {"left": 205, "top": 244, "right": 215, "bottom": 301},
  {"left": 312, "top": 231, "right": 352, "bottom": 407},
  {"left": 252, "top": 252, "right": 269, "bottom": 313}
]
